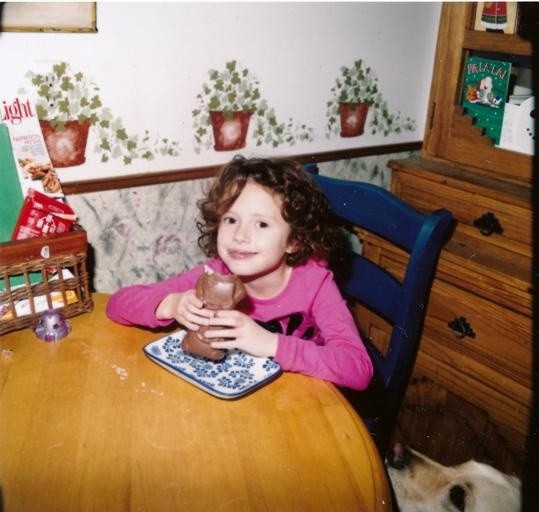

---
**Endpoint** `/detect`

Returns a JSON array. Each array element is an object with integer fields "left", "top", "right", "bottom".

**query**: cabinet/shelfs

[
  {"left": 349, "top": 156, "right": 531, "bottom": 450},
  {"left": 421, "top": 4, "right": 536, "bottom": 181}
]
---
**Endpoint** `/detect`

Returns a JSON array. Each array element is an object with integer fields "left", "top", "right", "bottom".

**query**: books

[{"left": 0, "top": 93, "right": 80, "bottom": 318}]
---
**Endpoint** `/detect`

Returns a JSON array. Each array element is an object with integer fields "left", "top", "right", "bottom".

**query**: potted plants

[
  {"left": 22, "top": 60, "right": 175, "bottom": 168},
  {"left": 188, "top": 59, "right": 313, "bottom": 151},
  {"left": 329, "top": 57, "right": 418, "bottom": 138}
]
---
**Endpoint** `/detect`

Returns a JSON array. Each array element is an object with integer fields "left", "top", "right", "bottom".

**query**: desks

[{"left": 2, "top": 291, "right": 397, "bottom": 512}]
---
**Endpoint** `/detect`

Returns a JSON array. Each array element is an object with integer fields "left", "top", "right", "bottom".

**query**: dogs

[{"left": 384, "top": 441, "right": 521, "bottom": 512}]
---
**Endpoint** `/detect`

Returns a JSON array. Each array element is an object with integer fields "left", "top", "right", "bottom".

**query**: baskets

[{"left": 0, "top": 224, "right": 94, "bottom": 336}]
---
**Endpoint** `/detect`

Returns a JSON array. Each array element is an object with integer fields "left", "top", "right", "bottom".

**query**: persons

[{"left": 106, "top": 154, "right": 375, "bottom": 393}]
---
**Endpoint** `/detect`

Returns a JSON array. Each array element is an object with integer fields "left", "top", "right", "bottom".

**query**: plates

[{"left": 141, "top": 329, "right": 284, "bottom": 397}]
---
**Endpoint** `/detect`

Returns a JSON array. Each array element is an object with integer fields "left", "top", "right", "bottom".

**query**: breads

[{"left": 182, "top": 266, "right": 246, "bottom": 362}]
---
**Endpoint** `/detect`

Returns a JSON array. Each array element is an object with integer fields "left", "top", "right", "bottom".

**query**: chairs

[{"left": 288, "top": 161, "right": 457, "bottom": 449}]
